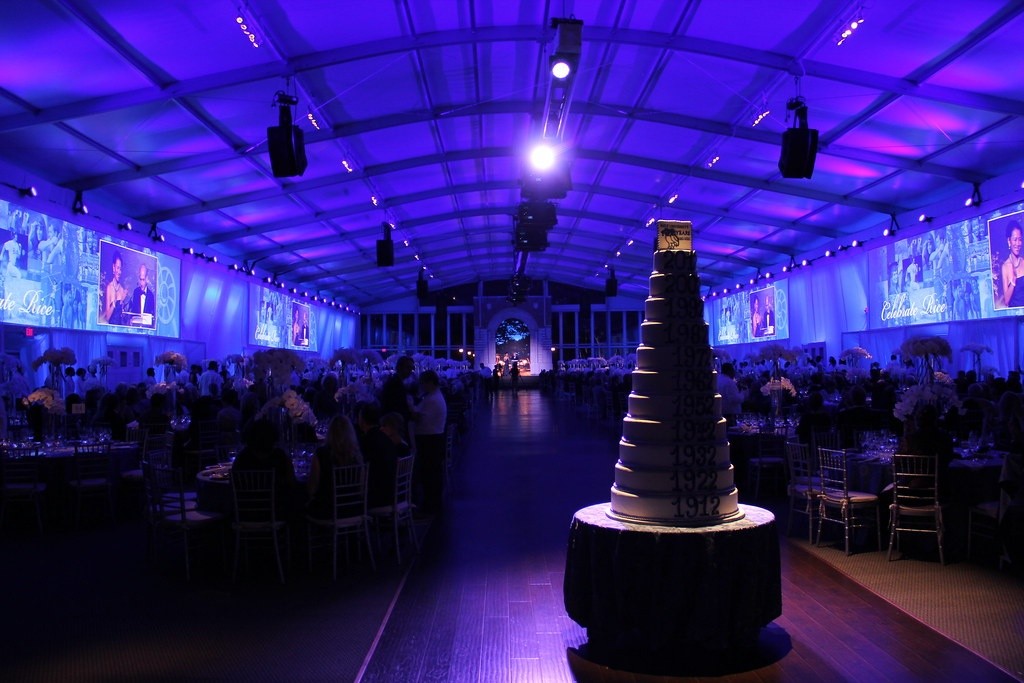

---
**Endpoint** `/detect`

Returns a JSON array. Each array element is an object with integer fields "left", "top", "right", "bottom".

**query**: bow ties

[
  {"left": 140, "top": 290, "right": 147, "bottom": 296},
  {"left": 303, "top": 326, "right": 306, "bottom": 329}
]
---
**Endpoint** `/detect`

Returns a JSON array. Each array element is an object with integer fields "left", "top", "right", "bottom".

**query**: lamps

[
  {"left": 18, "top": 187, "right": 361, "bottom": 317},
  {"left": 702, "top": 184, "right": 982, "bottom": 301}
]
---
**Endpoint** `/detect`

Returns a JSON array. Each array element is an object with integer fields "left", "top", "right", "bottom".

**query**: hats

[{"left": 420, "top": 370, "right": 439, "bottom": 384}]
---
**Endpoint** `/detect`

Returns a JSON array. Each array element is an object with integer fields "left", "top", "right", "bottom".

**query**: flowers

[
  {"left": 892, "top": 335, "right": 995, "bottom": 422},
  {"left": 329, "top": 346, "right": 472, "bottom": 411},
  {"left": 557, "top": 352, "right": 637, "bottom": 377},
  {"left": 712, "top": 343, "right": 873, "bottom": 398},
  {"left": 0, "top": 346, "right": 316, "bottom": 437}
]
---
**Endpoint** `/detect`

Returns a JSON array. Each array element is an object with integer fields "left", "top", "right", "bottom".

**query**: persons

[
  {"left": 510, "top": 352, "right": 518, "bottom": 373},
  {"left": 260, "top": 288, "right": 310, "bottom": 347},
  {"left": 887, "top": 211, "right": 1010, "bottom": 326},
  {"left": 479, "top": 361, "right": 634, "bottom": 420},
  {"left": 503, "top": 352, "right": 509, "bottom": 376},
  {"left": 1001, "top": 219, "right": 1024, "bottom": 307},
  {"left": 0, "top": 200, "right": 157, "bottom": 335},
  {"left": 752, "top": 295, "right": 775, "bottom": 337},
  {"left": 717, "top": 355, "right": 1024, "bottom": 438},
  {"left": 0, "top": 355, "right": 475, "bottom": 526}
]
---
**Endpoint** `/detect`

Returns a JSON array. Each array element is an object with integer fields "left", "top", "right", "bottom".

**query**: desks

[
  {"left": 563, "top": 503, "right": 780, "bottom": 655},
  {"left": 825, "top": 448, "right": 1009, "bottom": 549},
  {"left": 197, "top": 467, "right": 307, "bottom": 515},
  {"left": 725, "top": 426, "right": 796, "bottom": 450},
  {"left": 0, "top": 439, "right": 140, "bottom": 518}
]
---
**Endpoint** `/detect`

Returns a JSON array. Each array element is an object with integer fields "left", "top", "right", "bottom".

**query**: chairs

[
  {"left": 0, "top": 381, "right": 481, "bottom": 583},
  {"left": 540, "top": 378, "right": 1024, "bottom": 572}
]
